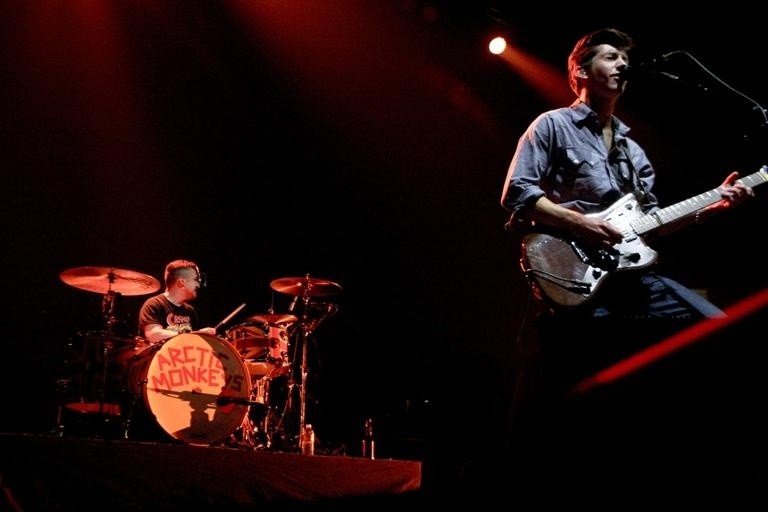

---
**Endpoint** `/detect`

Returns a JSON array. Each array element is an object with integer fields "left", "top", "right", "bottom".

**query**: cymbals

[
  {"left": 273, "top": 276, "right": 342, "bottom": 296},
  {"left": 245, "top": 312, "right": 298, "bottom": 327},
  {"left": 62, "top": 266, "right": 160, "bottom": 296}
]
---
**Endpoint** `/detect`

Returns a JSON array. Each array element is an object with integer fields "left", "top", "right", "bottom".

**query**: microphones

[{"left": 619, "top": 55, "right": 678, "bottom": 80}]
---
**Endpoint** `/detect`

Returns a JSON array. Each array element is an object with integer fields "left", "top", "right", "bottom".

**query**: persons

[
  {"left": 494, "top": 26, "right": 756, "bottom": 511},
  {"left": 134, "top": 257, "right": 245, "bottom": 449}
]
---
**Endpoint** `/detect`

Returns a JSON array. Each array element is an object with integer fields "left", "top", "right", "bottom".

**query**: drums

[
  {"left": 107, "top": 339, "right": 156, "bottom": 380},
  {"left": 121, "top": 330, "right": 253, "bottom": 447},
  {"left": 63, "top": 330, "right": 126, "bottom": 416},
  {"left": 248, "top": 375, "right": 273, "bottom": 406},
  {"left": 224, "top": 322, "right": 291, "bottom": 379}
]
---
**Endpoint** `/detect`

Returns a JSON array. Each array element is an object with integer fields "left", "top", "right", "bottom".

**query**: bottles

[
  {"left": 304, "top": 424, "right": 315, "bottom": 455},
  {"left": 362, "top": 419, "right": 377, "bottom": 460}
]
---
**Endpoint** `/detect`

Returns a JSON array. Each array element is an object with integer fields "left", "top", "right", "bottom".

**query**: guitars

[{"left": 520, "top": 164, "right": 767, "bottom": 314}]
